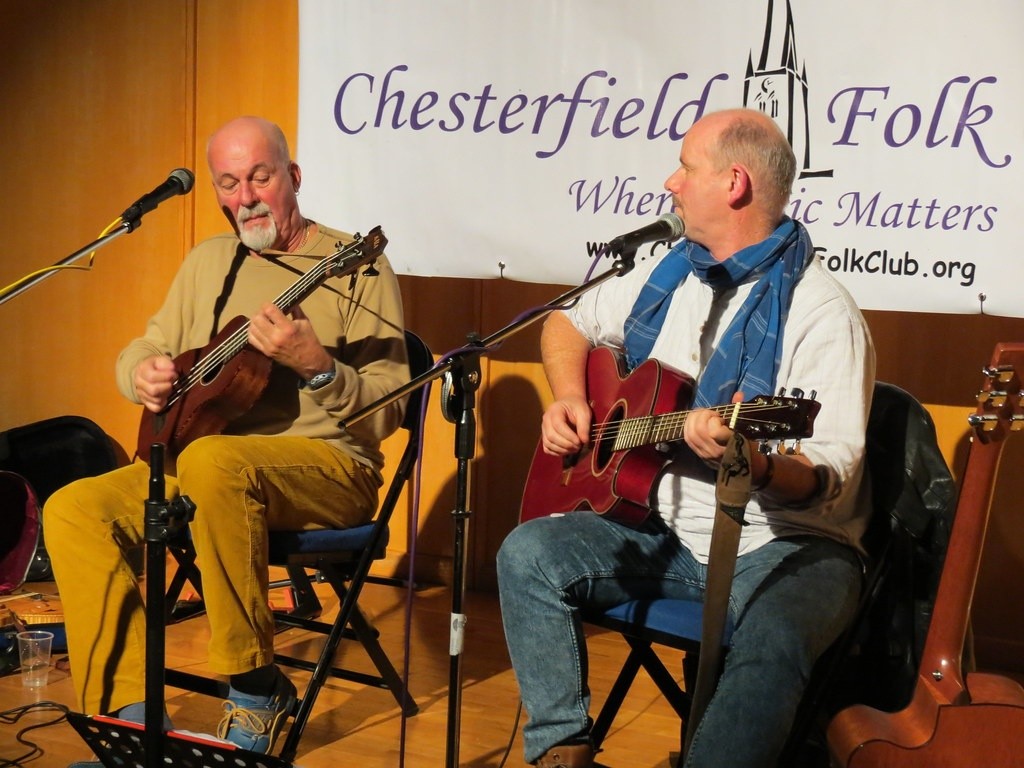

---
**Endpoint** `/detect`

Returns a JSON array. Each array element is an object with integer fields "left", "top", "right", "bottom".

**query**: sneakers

[{"left": 216, "top": 664, "right": 298, "bottom": 755}]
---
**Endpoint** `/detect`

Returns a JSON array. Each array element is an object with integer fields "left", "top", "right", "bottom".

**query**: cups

[{"left": 17, "top": 630, "right": 54, "bottom": 688}]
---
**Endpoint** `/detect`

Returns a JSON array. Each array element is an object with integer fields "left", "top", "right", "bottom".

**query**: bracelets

[{"left": 748, "top": 451, "right": 776, "bottom": 495}]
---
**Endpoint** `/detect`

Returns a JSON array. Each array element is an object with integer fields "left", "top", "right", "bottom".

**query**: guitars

[
  {"left": 518, "top": 339, "right": 824, "bottom": 531},
  {"left": 825, "top": 338, "right": 1024, "bottom": 768},
  {"left": 136, "top": 222, "right": 390, "bottom": 480}
]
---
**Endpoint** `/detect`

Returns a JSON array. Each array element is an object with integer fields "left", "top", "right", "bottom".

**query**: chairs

[
  {"left": 162, "top": 329, "right": 433, "bottom": 760},
  {"left": 569, "top": 379, "right": 958, "bottom": 768}
]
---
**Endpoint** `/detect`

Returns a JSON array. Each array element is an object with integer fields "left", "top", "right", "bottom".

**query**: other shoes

[{"left": 534, "top": 743, "right": 591, "bottom": 768}]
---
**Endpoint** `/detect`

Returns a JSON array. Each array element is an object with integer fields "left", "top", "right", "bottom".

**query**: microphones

[
  {"left": 121, "top": 169, "right": 196, "bottom": 221},
  {"left": 610, "top": 212, "right": 686, "bottom": 251}
]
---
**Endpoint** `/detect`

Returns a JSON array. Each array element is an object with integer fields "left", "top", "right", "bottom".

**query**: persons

[
  {"left": 42, "top": 116, "right": 411, "bottom": 756},
  {"left": 497, "top": 109, "right": 875, "bottom": 768}
]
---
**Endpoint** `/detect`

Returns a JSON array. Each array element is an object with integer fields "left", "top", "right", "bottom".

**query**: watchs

[{"left": 306, "top": 363, "right": 335, "bottom": 389}]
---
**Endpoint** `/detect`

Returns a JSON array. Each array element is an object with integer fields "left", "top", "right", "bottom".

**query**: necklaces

[{"left": 289, "top": 217, "right": 309, "bottom": 252}]
